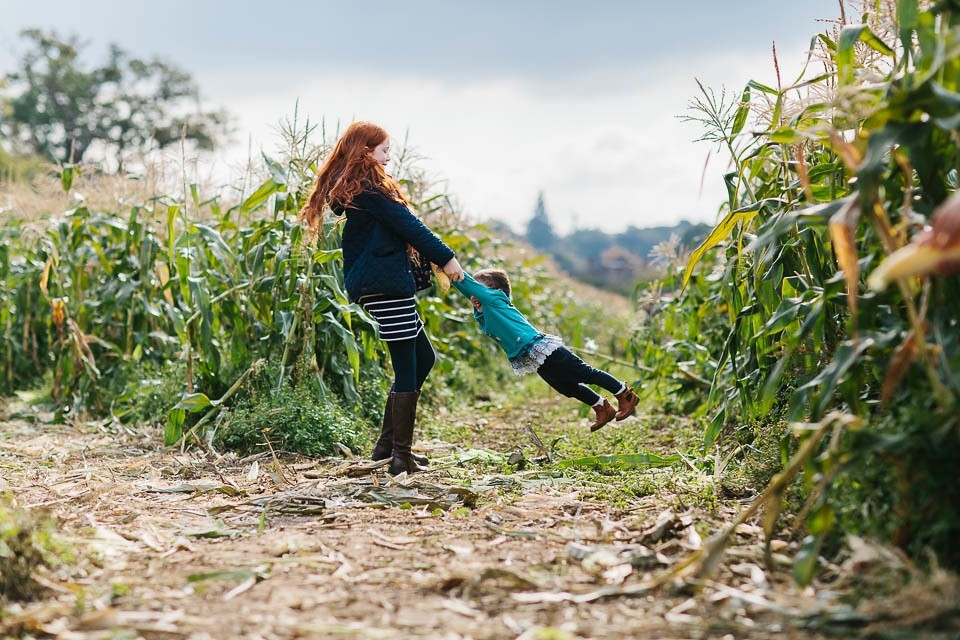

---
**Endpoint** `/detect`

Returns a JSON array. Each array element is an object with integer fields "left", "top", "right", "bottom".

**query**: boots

[
  {"left": 391, "top": 391, "right": 428, "bottom": 476},
  {"left": 614, "top": 381, "right": 640, "bottom": 421},
  {"left": 372, "top": 389, "right": 427, "bottom": 465},
  {"left": 591, "top": 399, "right": 616, "bottom": 432}
]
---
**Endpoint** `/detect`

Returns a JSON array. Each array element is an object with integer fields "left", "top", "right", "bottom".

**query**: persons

[
  {"left": 296, "top": 122, "right": 465, "bottom": 476},
  {"left": 452, "top": 267, "right": 640, "bottom": 431}
]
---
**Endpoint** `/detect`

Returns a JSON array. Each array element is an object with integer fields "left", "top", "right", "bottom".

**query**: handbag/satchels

[{"left": 410, "top": 254, "right": 432, "bottom": 291}]
